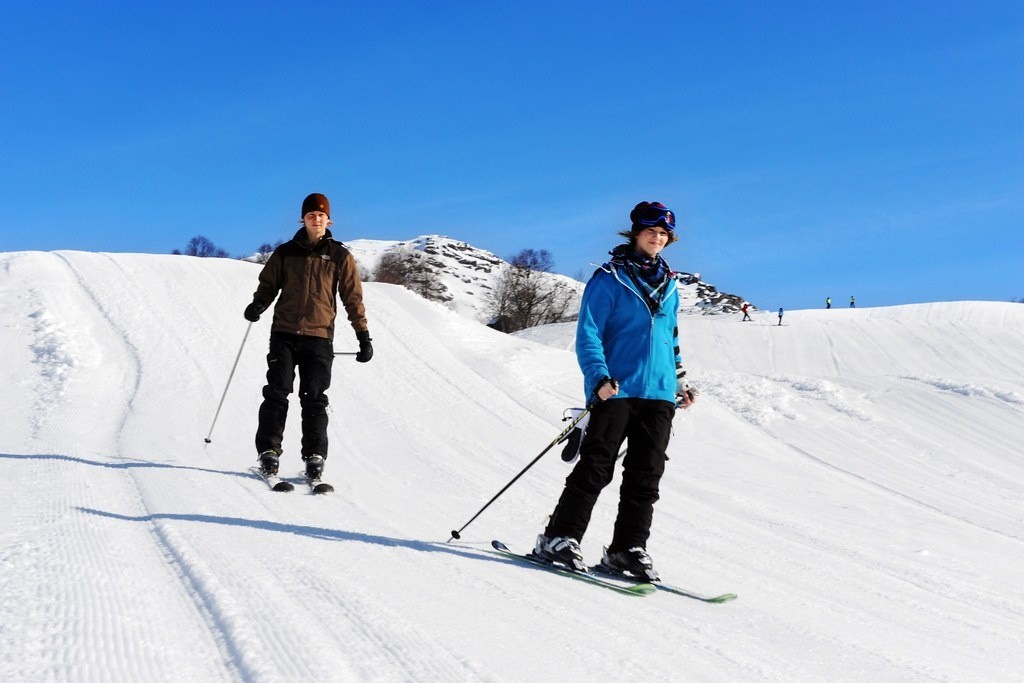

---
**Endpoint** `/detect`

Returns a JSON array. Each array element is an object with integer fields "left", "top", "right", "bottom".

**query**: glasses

[{"left": 639, "top": 206, "right": 675, "bottom": 229}]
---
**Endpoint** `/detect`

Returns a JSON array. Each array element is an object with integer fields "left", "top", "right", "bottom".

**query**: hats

[
  {"left": 630, "top": 200, "right": 676, "bottom": 232},
  {"left": 301, "top": 193, "right": 330, "bottom": 219}
]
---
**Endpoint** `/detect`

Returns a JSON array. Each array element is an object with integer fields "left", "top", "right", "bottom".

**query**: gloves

[
  {"left": 558, "top": 407, "right": 592, "bottom": 464},
  {"left": 244, "top": 301, "right": 266, "bottom": 323},
  {"left": 355, "top": 337, "right": 374, "bottom": 363}
]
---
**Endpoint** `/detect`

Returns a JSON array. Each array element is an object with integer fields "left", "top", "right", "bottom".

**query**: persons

[
  {"left": 741, "top": 303, "right": 751, "bottom": 321},
  {"left": 827, "top": 297, "right": 831, "bottom": 309},
  {"left": 244, "top": 192, "right": 373, "bottom": 480},
  {"left": 778, "top": 307, "right": 783, "bottom": 325},
  {"left": 850, "top": 296, "right": 855, "bottom": 307},
  {"left": 534, "top": 202, "right": 695, "bottom": 579}
]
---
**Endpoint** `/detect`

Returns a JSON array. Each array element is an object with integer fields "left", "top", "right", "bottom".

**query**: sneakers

[
  {"left": 534, "top": 533, "right": 584, "bottom": 572},
  {"left": 602, "top": 546, "right": 659, "bottom": 583},
  {"left": 306, "top": 454, "right": 325, "bottom": 479},
  {"left": 257, "top": 450, "right": 281, "bottom": 475}
]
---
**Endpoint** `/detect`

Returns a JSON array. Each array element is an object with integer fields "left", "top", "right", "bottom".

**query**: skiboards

[
  {"left": 491, "top": 538, "right": 740, "bottom": 607},
  {"left": 249, "top": 463, "right": 335, "bottom": 497}
]
